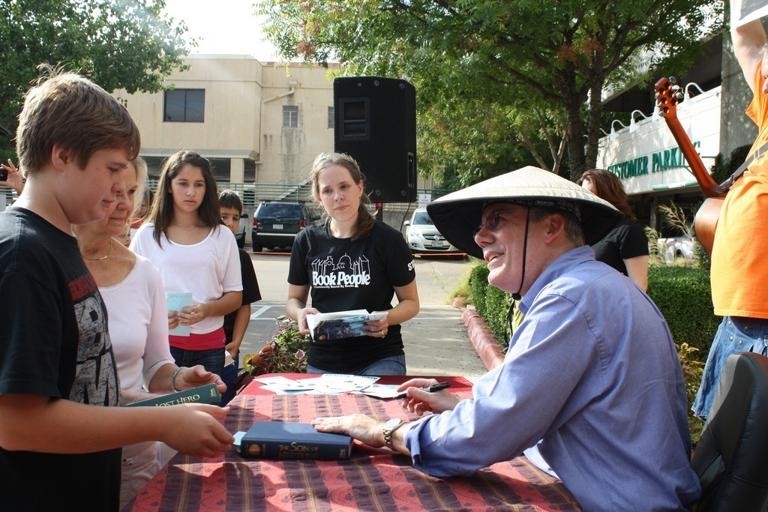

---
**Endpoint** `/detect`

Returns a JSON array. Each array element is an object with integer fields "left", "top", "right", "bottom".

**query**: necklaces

[{"left": 87, "top": 240, "right": 112, "bottom": 261}]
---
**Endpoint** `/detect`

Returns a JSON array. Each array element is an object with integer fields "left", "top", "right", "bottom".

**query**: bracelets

[{"left": 168, "top": 366, "right": 185, "bottom": 393}]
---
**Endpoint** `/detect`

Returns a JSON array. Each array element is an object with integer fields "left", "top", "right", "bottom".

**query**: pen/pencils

[{"left": 393, "top": 382, "right": 451, "bottom": 399}]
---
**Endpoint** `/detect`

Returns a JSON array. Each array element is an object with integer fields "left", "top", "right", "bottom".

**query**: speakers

[{"left": 333, "top": 76, "right": 416, "bottom": 202}]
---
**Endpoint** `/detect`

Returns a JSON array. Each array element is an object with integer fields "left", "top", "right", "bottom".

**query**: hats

[{"left": 426, "top": 165, "right": 625, "bottom": 259}]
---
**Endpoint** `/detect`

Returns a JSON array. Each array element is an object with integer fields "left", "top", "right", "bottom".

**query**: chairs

[{"left": 689, "top": 350, "right": 768, "bottom": 512}]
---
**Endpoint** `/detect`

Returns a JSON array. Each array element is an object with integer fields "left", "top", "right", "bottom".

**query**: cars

[
  {"left": 233, "top": 211, "right": 249, "bottom": 248},
  {"left": 404, "top": 208, "right": 468, "bottom": 257},
  {"left": 648, "top": 236, "right": 700, "bottom": 269}
]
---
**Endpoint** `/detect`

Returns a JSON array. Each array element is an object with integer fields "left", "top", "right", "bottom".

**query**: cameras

[{"left": 0, "top": 169, "right": 8, "bottom": 181}]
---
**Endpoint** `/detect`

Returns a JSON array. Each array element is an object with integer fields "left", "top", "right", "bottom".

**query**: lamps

[{"left": 595, "top": 82, "right": 706, "bottom": 141}]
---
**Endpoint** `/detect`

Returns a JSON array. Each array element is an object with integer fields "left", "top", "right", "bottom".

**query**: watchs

[{"left": 381, "top": 417, "right": 406, "bottom": 452}]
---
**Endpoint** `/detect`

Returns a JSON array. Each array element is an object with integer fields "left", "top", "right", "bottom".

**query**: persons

[
  {"left": 71, "top": 154, "right": 230, "bottom": 512},
  {"left": 126, "top": 149, "right": 243, "bottom": 408},
  {"left": 0, "top": 72, "right": 235, "bottom": 512},
  {"left": 113, "top": 223, "right": 130, "bottom": 248},
  {"left": 218, "top": 188, "right": 262, "bottom": 406},
  {"left": 573, "top": 168, "right": 650, "bottom": 294},
  {"left": 285, "top": 148, "right": 420, "bottom": 377},
  {"left": 127, "top": 186, "right": 154, "bottom": 240},
  {"left": 309, "top": 164, "right": 703, "bottom": 512},
  {"left": 0, "top": 157, "right": 25, "bottom": 193},
  {"left": 689, "top": 0, "right": 768, "bottom": 428}
]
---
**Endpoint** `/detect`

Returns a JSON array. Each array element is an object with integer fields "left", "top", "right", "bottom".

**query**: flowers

[{"left": 241, "top": 313, "right": 312, "bottom": 374}]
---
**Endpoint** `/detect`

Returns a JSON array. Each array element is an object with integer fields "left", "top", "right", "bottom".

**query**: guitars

[{"left": 653, "top": 77, "right": 736, "bottom": 256}]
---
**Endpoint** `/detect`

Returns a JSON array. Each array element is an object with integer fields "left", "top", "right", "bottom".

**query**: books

[
  {"left": 241, "top": 421, "right": 354, "bottom": 459},
  {"left": 305, "top": 307, "right": 383, "bottom": 343},
  {"left": 126, "top": 382, "right": 222, "bottom": 407}
]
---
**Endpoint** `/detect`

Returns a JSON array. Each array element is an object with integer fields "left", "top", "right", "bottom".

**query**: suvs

[{"left": 252, "top": 198, "right": 321, "bottom": 252}]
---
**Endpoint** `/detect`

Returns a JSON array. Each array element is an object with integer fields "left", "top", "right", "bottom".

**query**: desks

[{"left": 120, "top": 373, "right": 583, "bottom": 512}]
